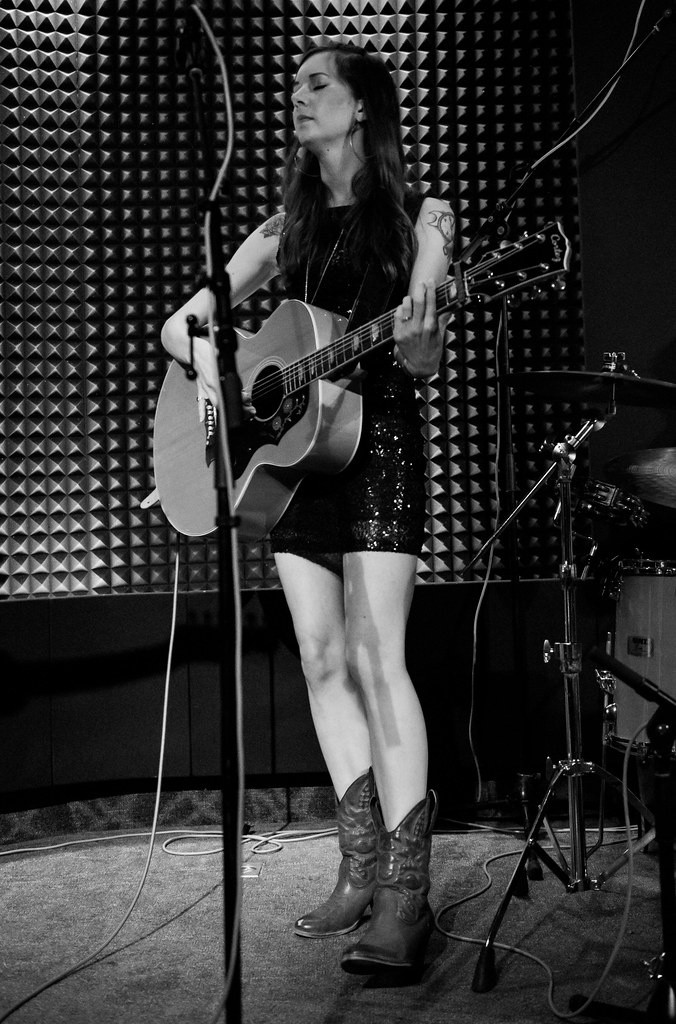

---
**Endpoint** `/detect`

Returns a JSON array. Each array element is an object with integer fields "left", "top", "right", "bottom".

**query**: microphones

[{"left": 175, "top": 4, "right": 210, "bottom": 75}]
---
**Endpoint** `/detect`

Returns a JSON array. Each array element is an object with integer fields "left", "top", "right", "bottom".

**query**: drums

[{"left": 607, "top": 558, "right": 676, "bottom": 759}]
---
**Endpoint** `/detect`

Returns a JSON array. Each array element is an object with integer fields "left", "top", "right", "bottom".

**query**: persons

[{"left": 161, "top": 41, "right": 456, "bottom": 977}]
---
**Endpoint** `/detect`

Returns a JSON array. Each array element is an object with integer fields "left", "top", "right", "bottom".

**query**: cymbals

[
  {"left": 498, "top": 367, "right": 676, "bottom": 408},
  {"left": 603, "top": 447, "right": 676, "bottom": 508},
  {"left": 554, "top": 474, "right": 650, "bottom": 527}
]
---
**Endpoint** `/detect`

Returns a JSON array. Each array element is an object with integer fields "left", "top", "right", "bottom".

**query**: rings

[
  {"left": 196, "top": 396, "right": 205, "bottom": 402},
  {"left": 401, "top": 316, "right": 411, "bottom": 323}
]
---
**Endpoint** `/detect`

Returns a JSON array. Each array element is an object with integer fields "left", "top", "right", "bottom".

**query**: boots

[
  {"left": 294, "top": 766, "right": 377, "bottom": 939},
  {"left": 341, "top": 789, "right": 437, "bottom": 974}
]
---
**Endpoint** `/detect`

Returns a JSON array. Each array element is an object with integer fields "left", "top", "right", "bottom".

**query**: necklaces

[{"left": 305, "top": 219, "right": 348, "bottom": 306}]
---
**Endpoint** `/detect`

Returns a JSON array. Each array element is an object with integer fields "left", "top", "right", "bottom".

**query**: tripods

[{"left": 460, "top": 381, "right": 657, "bottom": 994}]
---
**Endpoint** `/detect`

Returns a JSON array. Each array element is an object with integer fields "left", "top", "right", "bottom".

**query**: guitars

[{"left": 152, "top": 220, "right": 573, "bottom": 539}]
